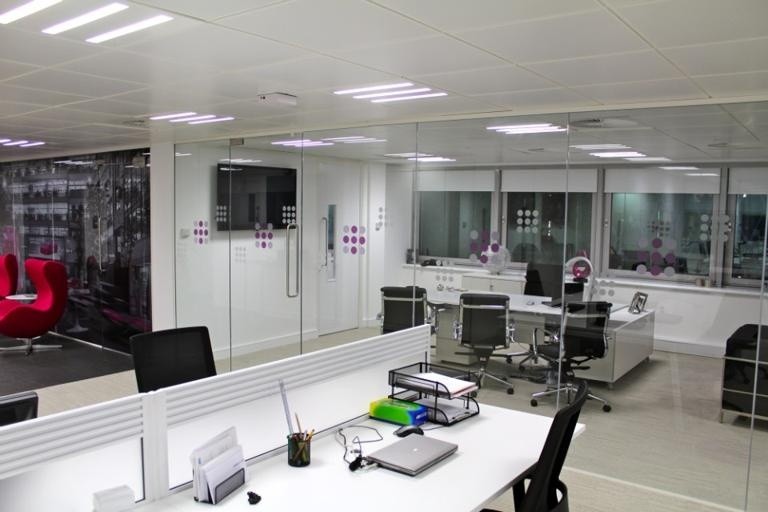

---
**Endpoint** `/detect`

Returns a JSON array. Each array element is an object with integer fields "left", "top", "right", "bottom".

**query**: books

[
  {"left": 416, "top": 397, "right": 472, "bottom": 423},
  {"left": 402, "top": 370, "right": 478, "bottom": 399},
  {"left": 189, "top": 425, "right": 239, "bottom": 502},
  {"left": 200, "top": 444, "right": 248, "bottom": 505}
]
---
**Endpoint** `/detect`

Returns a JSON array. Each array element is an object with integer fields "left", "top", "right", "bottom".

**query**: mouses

[
  {"left": 527, "top": 300, "right": 534, "bottom": 306},
  {"left": 394, "top": 425, "right": 424, "bottom": 437}
]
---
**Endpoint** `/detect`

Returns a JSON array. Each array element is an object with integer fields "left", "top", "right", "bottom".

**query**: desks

[
  {"left": 426, "top": 286, "right": 656, "bottom": 390},
  {"left": 168, "top": 395, "right": 584, "bottom": 511}
]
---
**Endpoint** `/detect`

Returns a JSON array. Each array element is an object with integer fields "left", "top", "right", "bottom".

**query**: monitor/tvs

[
  {"left": 0, "top": 391, "right": 38, "bottom": 426},
  {"left": 217, "top": 163, "right": 297, "bottom": 231}
]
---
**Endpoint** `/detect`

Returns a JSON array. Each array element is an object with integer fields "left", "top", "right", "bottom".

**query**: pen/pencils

[{"left": 289, "top": 411, "right": 315, "bottom": 466}]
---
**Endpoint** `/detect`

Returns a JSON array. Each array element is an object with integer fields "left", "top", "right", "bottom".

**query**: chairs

[
  {"left": 0, "top": 254, "right": 68, "bottom": 355},
  {"left": 480, "top": 379, "right": 588, "bottom": 512},
  {"left": 129, "top": 326, "right": 217, "bottom": 392},
  {"left": 377, "top": 261, "right": 613, "bottom": 412}
]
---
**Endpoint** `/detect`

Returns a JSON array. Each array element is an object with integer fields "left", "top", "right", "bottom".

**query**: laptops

[
  {"left": 366, "top": 432, "right": 458, "bottom": 476},
  {"left": 542, "top": 282, "right": 584, "bottom": 307}
]
